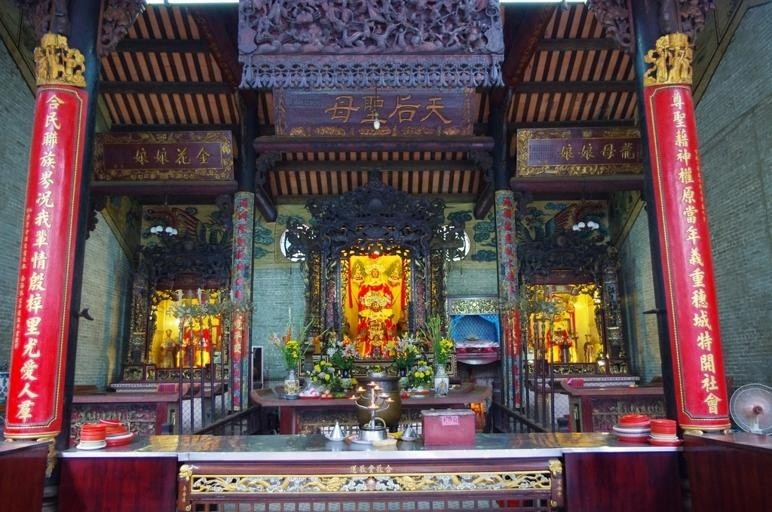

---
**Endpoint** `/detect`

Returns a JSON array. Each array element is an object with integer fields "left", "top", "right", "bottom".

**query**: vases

[
  {"left": 304, "top": 332, "right": 357, "bottom": 392},
  {"left": 385, "top": 331, "right": 433, "bottom": 388},
  {"left": 419, "top": 312, "right": 454, "bottom": 362},
  {"left": 267, "top": 306, "right": 327, "bottom": 370}
]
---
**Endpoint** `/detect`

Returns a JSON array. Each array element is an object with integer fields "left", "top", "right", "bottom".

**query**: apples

[{"left": 349, "top": 380, "right": 395, "bottom": 410}]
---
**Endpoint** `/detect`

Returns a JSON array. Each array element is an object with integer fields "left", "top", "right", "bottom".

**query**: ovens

[{"left": 454, "top": 339, "right": 501, "bottom": 377}]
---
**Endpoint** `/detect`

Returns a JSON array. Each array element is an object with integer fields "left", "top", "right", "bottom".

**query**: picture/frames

[
  {"left": 560, "top": 378, "right": 667, "bottom": 432},
  {"left": 249, "top": 383, "right": 492, "bottom": 435},
  {"left": 71, "top": 384, "right": 229, "bottom": 436}
]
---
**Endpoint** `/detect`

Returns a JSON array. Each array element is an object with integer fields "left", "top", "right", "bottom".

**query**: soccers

[
  {"left": 322, "top": 390, "right": 332, "bottom": 398},
  {"left": 298, "top": 387, "right": 318, "bottom": 396}
]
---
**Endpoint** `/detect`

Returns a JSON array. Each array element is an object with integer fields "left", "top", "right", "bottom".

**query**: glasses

[
  {"left": 572, "top": 194, "right": 600, "bottom": 234},
  {"left": 149, "top": 194, "right": 178, "bottom": 238}
]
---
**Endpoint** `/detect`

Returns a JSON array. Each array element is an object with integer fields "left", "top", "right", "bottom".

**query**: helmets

[
  {"left": 400, "top": 435, "right": 418, "bottom": 442},
  {"left": 324, "top": 434, "right": 346, "bottom": 442}
]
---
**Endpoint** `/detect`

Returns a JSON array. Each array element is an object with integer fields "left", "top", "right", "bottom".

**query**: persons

[
  {"left": 357, "top": 300, "right": 394, "bottom": 358},
  {"left": 583, "top": 335, "right": 595, "bottom": 363},
  {"left": 159, "top": 328, "right": 177, "bottom": 368},
  {"left": 546, "top": 314, "right": 578, "bottom": 364},
  {"left": 363, "top": 335, "right": 386, "bottom": 359},
  {"left": 183, "top": 318, "right": 211, "bottom": 366},
  {"left": 358, "top": 268, "right": 394, "bottom": 347}
]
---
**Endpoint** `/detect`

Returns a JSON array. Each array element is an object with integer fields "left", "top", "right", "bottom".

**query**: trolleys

[{"left": 730, "top": 383, "right": 772, "bottom": 435}]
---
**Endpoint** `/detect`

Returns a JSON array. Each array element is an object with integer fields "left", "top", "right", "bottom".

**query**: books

[{"left": 420, "top": 408, "right": 476, "bottom": 446}]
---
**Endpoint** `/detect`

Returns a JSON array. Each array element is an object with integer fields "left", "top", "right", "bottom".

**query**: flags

[
  {"left": 283, "top": 368, "right": 299, "bottom": 394},
  {"left": 433, "top": 364, "right": 449, "bottom": 397},
  {"left": 397, "top": 369, "right": 428, "bottom": 399}
]
